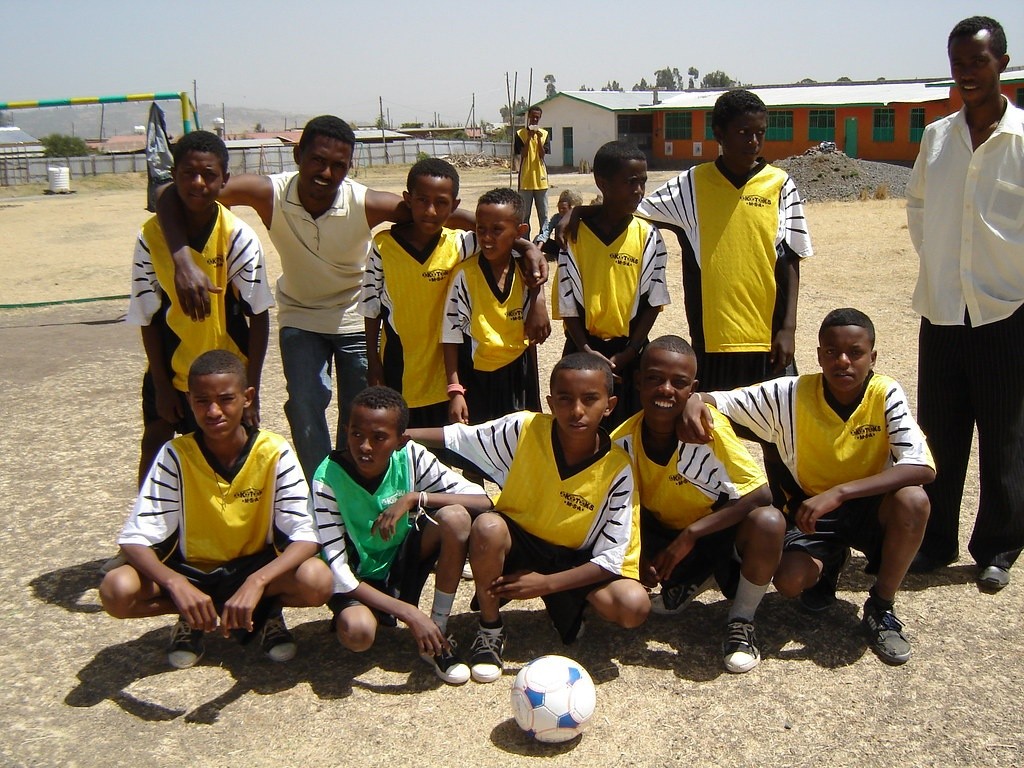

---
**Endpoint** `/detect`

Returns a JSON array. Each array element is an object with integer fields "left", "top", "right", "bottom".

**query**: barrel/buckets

[{"left": 48, "top": 167, "right": 70, "bottom": 193}]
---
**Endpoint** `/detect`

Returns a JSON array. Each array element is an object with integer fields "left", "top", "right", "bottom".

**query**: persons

[
  {"left": 127, "top": 116, "right": 551, "bottom": 490},
  {"left": 865, "top": 16, "right": 1024, "bottom": 588},
  {"left": 609, "top": 336, "right": 787, "bottom": 672},
  {"left": 403, "top": 354, "right": 650, "bottom": 682},
  {"left": 312, "top": 385, "right": 494, "bottom": 685},
  {"left": 99, "top": 350, "right": 335, "bottom": 668},
  {"left": 535, "top": 89, "right": 811, "bottom": 430},
  {"left": 679, "top": 308, "right": 936, "bottom": 664},
  {"left": 513, "top": 106, "right": 551, "bottom": 243}
]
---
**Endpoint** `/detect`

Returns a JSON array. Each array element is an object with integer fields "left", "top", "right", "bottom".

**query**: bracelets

[
  {"left": 694, "top": 392, "right": 702, "bottom": 401},
  {"left": 415, "top": 491, "right": 439, "bottom": 531}
]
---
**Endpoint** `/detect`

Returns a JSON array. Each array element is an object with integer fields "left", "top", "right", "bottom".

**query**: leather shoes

[
  {"left": 909, "top": 553, "right": 959, "bottom": 572},
  {"left": 977, "top": 566, "right": 1010, "bottom": 588}
]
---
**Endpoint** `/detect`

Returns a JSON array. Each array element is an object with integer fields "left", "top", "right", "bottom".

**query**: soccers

[{"left": 511, "top": 653, "right": 596, "bottom": 741}]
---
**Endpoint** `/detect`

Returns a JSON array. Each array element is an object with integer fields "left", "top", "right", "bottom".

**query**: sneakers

[
  {"left": 801, "top": 547, "right": 852, "bottom": 611},
  {"left": 721, "top": 617, "right": 761, "bottom": 673},
  {"left": 650, "top": 572, "right": 717, "bottom": 615},
  {"left": 102, "top": 547, "right": 130, "bottom": 575},
  {"left": 167, "top": 615, "right": 206, "bottom": 669},
  {"left": 861, "top": 598, "right": 911, "bottom": 664},
  {"left": 470, "top": 623, "right": 507, "bottom": 682},
  {"left": 419, "top": 634, "right": 471, "bottom": 684},
  {"left": 253, "top": 613, "right": 298, "bottom": 661}
]
